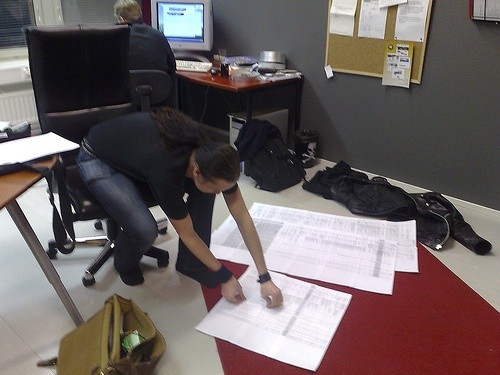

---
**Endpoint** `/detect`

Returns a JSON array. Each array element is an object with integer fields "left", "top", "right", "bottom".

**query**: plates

[{"left": 225, "top": 56, "right": 259, "bottom": 65}]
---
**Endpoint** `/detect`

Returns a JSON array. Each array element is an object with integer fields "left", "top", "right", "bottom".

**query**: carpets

[{"left": 199, "top": 243, "right": 500, "bottom": 375}]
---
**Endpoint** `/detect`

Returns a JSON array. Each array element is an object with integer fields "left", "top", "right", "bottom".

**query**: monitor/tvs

[{"left": 150, "top": 0, "right": 214, "bottom": 63}]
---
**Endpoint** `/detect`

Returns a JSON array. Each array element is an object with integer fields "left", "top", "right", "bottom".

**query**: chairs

[
  {"left": 130, "top": 70, "right": 174, "bottom": 112},
  {"left": 22, "top": 24, "right": 168, "bottom": 287}
]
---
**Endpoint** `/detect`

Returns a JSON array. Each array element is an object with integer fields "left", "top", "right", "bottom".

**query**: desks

[
  {"left": 0, "top": 153, "right": 84, "bottom": 327},
  {"left": 176, "top": 70, "right": 304, "bottom": 150}
]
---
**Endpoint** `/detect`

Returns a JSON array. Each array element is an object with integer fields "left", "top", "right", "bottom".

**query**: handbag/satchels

[
  {"left": 55, "top": 293, "right": 166, "bottom": 375},
  {"left": 0, "top": 155, "right": 53, "bottom": 176}
]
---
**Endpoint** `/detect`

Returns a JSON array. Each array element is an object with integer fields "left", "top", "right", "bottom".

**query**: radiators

[{"left": 0, "top": 89, "right": 40, "bottom": 130}]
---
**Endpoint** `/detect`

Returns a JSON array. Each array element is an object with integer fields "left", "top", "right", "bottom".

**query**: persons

[
  {"left": 76, "top": 109, "right": 283, "bottom": 307},
  {"left": 111, "top": 0, "right": 176, "bottom": 107}
]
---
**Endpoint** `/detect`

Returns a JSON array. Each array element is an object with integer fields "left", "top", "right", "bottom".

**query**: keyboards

[{"left": 174, "top": 59, "right": 213, "bottom": 73}]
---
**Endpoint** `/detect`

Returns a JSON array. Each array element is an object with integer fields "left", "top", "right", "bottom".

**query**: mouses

[{"left": 211, "top": 67, "right": 219, "bottom": 75}]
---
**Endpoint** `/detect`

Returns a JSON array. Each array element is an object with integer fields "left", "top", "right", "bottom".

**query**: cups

[{"left": 221, "top": 63, "right": 229, "bottom": 78}]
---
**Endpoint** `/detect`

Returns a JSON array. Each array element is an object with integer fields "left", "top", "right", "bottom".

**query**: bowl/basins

[
  {"left": 259, "top": 51, "right": 286, "bottom": 64},
  {"left": 232, "top": 71, "right": 260, "bottom": 80}
]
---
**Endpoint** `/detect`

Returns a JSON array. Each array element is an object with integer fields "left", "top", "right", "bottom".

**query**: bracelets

[
  {"left": 257, "top": 272, "right": 271, "bottom": 283},
  {"left": 207, "top": 264, "right": 233, "bottom": 284}
]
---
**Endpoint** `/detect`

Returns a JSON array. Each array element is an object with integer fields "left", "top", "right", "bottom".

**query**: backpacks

[{"left": 249, "top": 136, "right": 306, "bottom": 192}]
